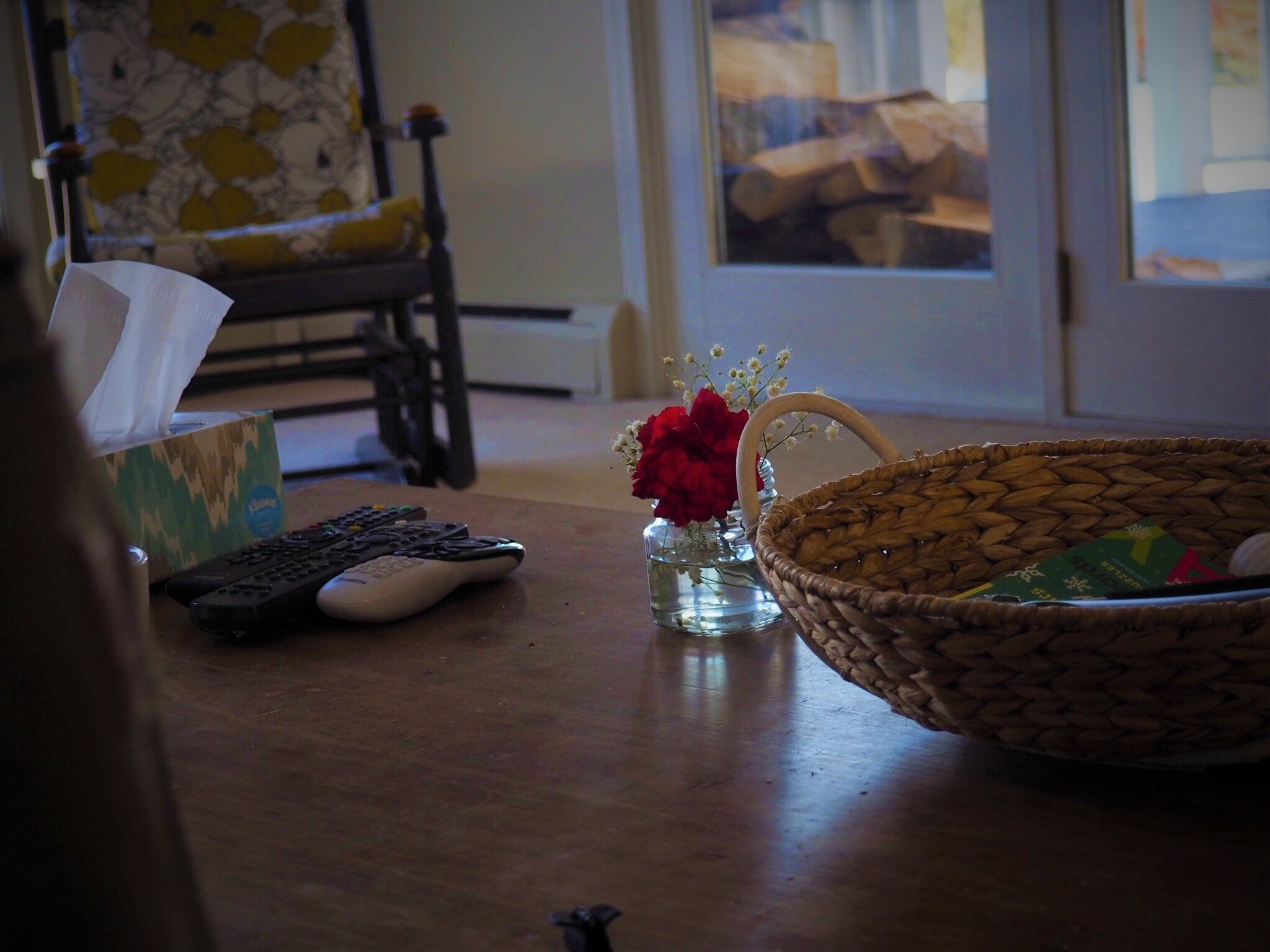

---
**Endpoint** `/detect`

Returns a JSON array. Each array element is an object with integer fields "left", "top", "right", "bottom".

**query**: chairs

[
  {"left": 0, "top": 222, "right": 214, "bottom": 952},
  {"left": 21, "top": 0, "right": 475, "bottom": 493}
]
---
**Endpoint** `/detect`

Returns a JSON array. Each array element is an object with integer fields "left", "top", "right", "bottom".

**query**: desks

[{"left": 149, "top": 478, "right": 1270, "bottom": 952}]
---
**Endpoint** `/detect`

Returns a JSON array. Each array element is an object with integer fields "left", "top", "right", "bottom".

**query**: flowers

[{"left": 610, "top": 344, "right": 848, "bottom": 626}]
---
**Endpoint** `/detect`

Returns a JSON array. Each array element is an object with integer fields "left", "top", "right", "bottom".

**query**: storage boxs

[{"left": 711, "top": 32, "right": 992, "bottom": 269}]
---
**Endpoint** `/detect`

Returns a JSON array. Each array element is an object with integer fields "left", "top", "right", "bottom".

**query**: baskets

[{"left": 736, "top": 391, "right": 1270, "bottom": 771}]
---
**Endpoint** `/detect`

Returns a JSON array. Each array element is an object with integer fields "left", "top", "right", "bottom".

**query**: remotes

[
  {"left": 317, "top": 535, "right": 526, "bottom": 622},
  {"left": 190, "top": 520, "right": 470, "bottom": 637},
  {"left": 164, "top": 504, "right": 428, "bottom": 608}
]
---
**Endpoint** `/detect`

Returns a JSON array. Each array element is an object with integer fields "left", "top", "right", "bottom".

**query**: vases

[{"left": 643, "top": 459, "right": 787, "bottom": 636}]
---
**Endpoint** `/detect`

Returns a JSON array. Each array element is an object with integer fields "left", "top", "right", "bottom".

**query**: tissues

[{"left": 42, "top": 259, "right": 291, "bottom": 593}]
